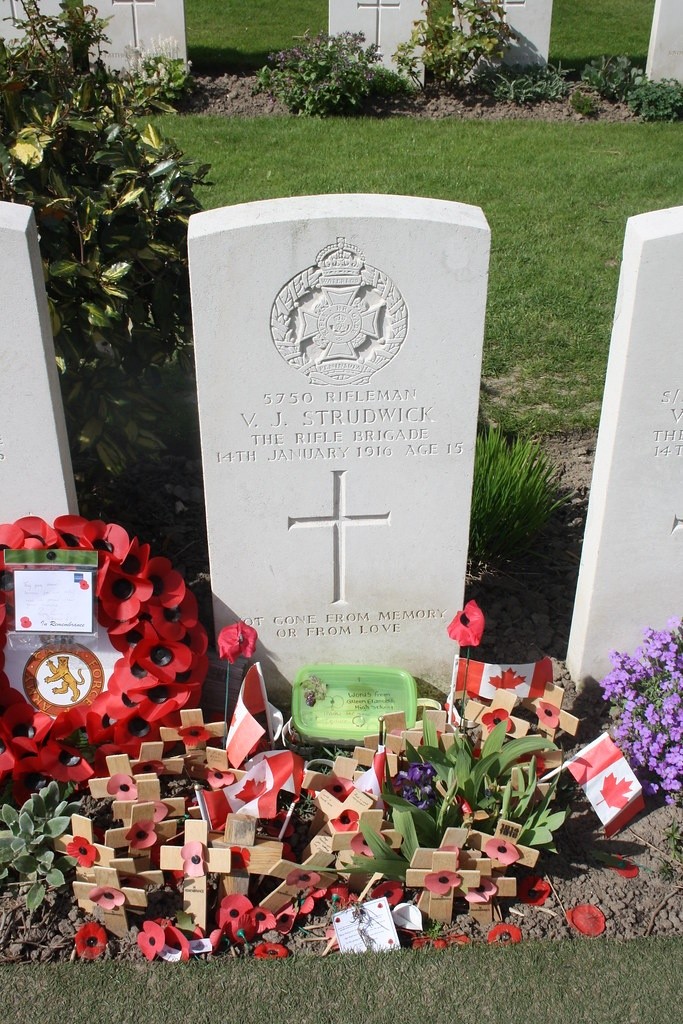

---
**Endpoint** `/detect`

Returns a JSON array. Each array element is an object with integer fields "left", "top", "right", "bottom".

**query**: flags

[
  {"left": 204, "top": 750, "right": 294, "bottom": 830},
  {"left": 454, "top": 658, "right": 553, "bottom": 699},
  {"left": 226, "top": 664, "right": 265, "bottom": 770},
  {"left": 335, "top": 751, "right": 390, "bottom": 811},
  {"left": 566, "top": 737, "right": 646, "bottom": 838},
  {"left": 240, "top": 750, "right": 304, "bottom": 802}
]
